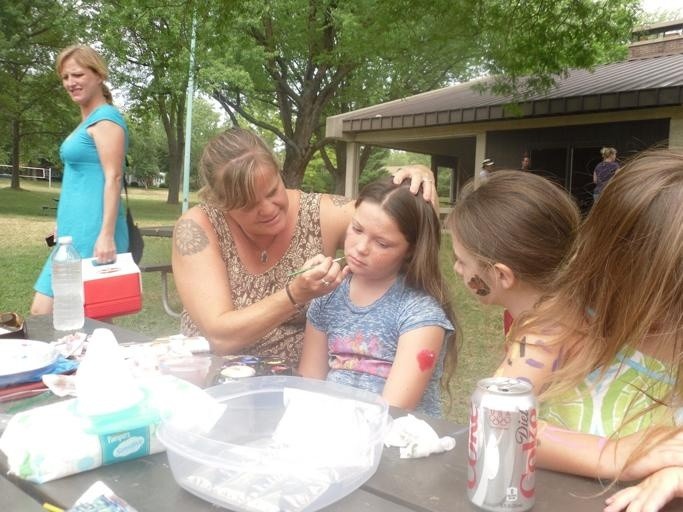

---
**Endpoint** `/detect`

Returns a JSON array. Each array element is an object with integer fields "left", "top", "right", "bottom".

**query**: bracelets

[{"left": 286, "top": 284, "right": 304, "bottom": 310}]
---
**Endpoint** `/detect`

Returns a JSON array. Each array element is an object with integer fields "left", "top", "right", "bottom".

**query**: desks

[{"left": 0, "top": 312, "right": 683, "bottom": 512}]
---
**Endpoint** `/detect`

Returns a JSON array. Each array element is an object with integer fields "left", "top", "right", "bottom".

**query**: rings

[
  {"left": 423, "top": 176, "right": 433, "bottom": 182},
  {"left": 321, "top": 278, "right": 330, "bottom": 287}
]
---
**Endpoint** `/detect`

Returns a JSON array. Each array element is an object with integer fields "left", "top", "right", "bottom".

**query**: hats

[{"left": 481, "top": 158, "right": 495, "bottom": 168}]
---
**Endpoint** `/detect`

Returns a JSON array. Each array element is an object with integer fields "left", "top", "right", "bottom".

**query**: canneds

[{"left": 466, "top": 376, "right": 539, "bottom": 511}]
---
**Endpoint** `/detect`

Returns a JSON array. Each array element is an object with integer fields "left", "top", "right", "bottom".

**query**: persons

[
  {"left": 479, "top": 159, "right": 495, "bottom": 185},
  {"left": 30, "top": 45, "right": 130, "bottom": 324},
  {"left": 521, "top": 156, "right": 534, "bottom": 174},
  {"left": 592, "top": 147, "right": 620, "bottom": 203},
  {"left": 533, "top": 149, "right": 683, "bottom": 512},
  {"left": 443, "top": 168, "right": 603, "bottom": 398},
  {"left": 299, "top": 173, "right": 462, "bottom": 419},
  {"left": 172, "top": 127, "right": 440, "bottom": 374}
]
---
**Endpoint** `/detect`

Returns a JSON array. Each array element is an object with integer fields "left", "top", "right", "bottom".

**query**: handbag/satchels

[{"left": 123, "top": 207, "right": 144, "bottom": 263}]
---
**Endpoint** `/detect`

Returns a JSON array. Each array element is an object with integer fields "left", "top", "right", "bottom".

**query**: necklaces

[{"left": 243, "top": 232, "right": 279, "bottom": 265}]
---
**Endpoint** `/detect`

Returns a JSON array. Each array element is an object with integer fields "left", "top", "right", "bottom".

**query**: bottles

[{"left": 52, "top": 233, "right": 85, "bottom": 332}]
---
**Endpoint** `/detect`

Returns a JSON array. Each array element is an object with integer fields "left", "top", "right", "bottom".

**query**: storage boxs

[
  {"left": 157, "top": 369, "right": 391, "bottom": 508},
  {"left": 78, "top": 252, "right": 144, "bottom": 319}
]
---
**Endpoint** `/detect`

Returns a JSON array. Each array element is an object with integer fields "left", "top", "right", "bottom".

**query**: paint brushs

[{"left": 287, "top": 256, "right": 345, "bottom": 278}]
